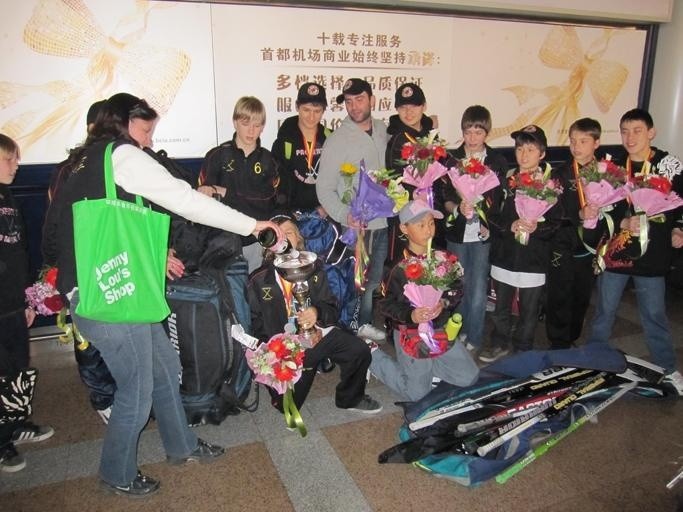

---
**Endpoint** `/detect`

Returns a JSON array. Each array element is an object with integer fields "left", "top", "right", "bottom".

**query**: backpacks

[
  {"left": 143, "top": 259, "right": 252, "bottom": 426},
  {"left": 292, "top": 213, "right": 357, "bottom": 328},
  {"left": 141, "top": 144, "right": 245, "bottom": 269}
]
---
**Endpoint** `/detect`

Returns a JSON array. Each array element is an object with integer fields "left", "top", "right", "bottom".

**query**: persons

[
  {"left": 545, "top": 119, "right": 606, "bottom": 349},
  {"left": 589, "top": 109, "right": 683, "bottom": 375},
  {"left": 198, "top": 97, "right": 281, "bottom": 223},
  {"left": 271, "top": 83, "right": 354, "bottom": 326},
  {"left": 361, "top": 200, "right": 478, "bottom": 402},
  {"left": 55, "top": 94, "right": 286, "bottom": 496},
  {"left": 478, "top": 125, "right": 559, "bottom": 362},
  {"left": 246, "top": 211, "right": 383, "bottom": 415},
  {"left": 386, "top": 84, "right": 445, "bottom": 266},
  {"left": 0, "top": 134, "right": 54, "bottom": 473},
  {"left": 49, "top": 101, "right": 117, "bottom": 425},
  {"left": 316, "top": 79, "right": 391, "bottom": 342},
  {"left": 443, "top": 106, "right": 508, "bottom": 351}
]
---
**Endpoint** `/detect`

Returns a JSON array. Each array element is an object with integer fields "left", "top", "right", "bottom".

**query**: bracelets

[{"left": 210, "top": 184, "right": 218, "bottom": 193}]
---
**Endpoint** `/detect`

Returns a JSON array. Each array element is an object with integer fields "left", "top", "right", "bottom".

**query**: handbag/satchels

[{"left": 70, "top": 198, "right": 171, "bottom": 323}]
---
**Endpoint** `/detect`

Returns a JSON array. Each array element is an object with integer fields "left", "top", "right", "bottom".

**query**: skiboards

[{"left": 378, "top": 357, "right": 667, "bottom": 483}]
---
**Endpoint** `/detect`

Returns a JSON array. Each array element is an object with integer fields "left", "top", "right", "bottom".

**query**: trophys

[{"left": 273, "top": 251, "right": 321, "bottom": 349}]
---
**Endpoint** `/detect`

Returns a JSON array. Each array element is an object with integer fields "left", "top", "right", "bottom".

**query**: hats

[
  {"left": 297, "top": 82, "right": 327, "bottom": 105},
  {"left": 399, "top": 200, "right": 444, "bottom": 225},
  {"left": 336, "top": 78, "right": 372, "bottom": 104},
  {"left": 394, "top": 83, "right": 425, "bottom": 108},
  {"left": 511, "top": 124, "right": 547, "bottom": 144}
]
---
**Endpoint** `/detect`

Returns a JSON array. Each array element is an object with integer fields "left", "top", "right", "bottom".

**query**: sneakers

[
  {"left": 0, "top": 444, "right": 26, "bottom": 474},
  {"left": 358, "top": 323, "right": 387, "bottom": 353},
  {"left": 346, "top": 396, "right": 383, "bottom": 414},
  {"left": 666, "top": 371, "right": 683, "bottom": 397},
  {"left": 96, "top": 405, "right": 113, "bottom": 425},
  {"left": 166, "top": 436, "right": 226, "bottom": 467},
  {"left": 103, "top": 470, "right": 161, "bottom": 499},
  {"left": 10, "top": 424, "right": 54, "bottom": 445},
  {"left": 479, "top": 341, "right": 510, "bottom": 362}
]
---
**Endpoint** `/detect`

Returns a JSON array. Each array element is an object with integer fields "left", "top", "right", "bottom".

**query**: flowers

[
  {"left": 577, "top": 160, "right": 630, "bottom": 229},
  {"left": 246, "top": 333, "right": 309, "bottom": 440},
  {"left": 400, "top": 139, "right": 450, "bottom": 201},
  {"left": 339, "top": 161, "right": 410, "bottom": 246},
  {"left": 624, "top": 175, "right": 683, "bottom": 236},
  {"left": 508, "top": 161, "right": 564, "bottom": 244},
  {"left": 398, "top": 249, "right": 464, "bottom": 333},
  {"left": 447, "top": 155, "right": 500, "bottom": 219},
  {"left": 24, "top": 263, "right": 89, "bottom": 351}
]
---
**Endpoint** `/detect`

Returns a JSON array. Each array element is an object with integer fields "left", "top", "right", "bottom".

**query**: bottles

[
  {"left": 442, "top": 312, "right": 463, "bottom": 343},
  {"left": 257, "top": 227, "right": 300, "bottom": 260}
]
